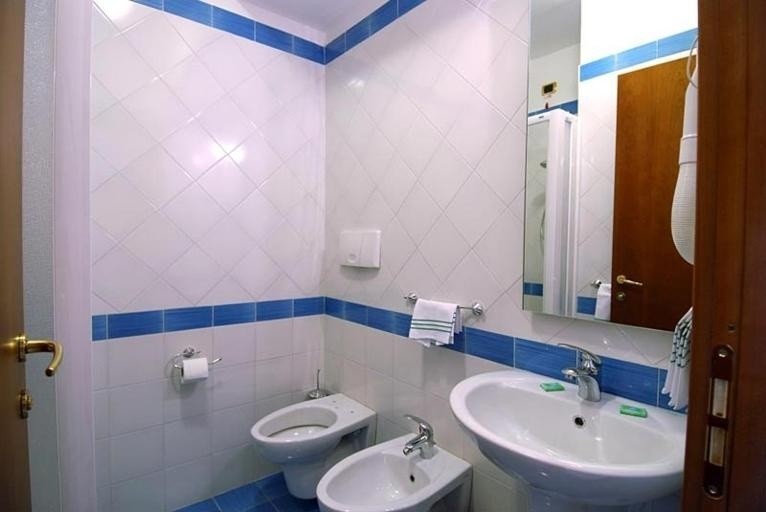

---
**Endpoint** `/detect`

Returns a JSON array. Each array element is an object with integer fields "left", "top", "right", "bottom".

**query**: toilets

[{"left": 249, "top": 394, "right": 377, "bottom": 499}]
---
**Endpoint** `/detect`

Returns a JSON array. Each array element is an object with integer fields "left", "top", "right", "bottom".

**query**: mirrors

[{"left": 519, "top": 0, "right": 698, "bottom": 337}]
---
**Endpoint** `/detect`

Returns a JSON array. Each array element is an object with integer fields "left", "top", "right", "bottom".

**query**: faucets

[
  {"left": 401, "top": 412, "right": 437, "bottom": 461},
  {"left": 560, "top": 340, "right": 605, "bottom": 403}
]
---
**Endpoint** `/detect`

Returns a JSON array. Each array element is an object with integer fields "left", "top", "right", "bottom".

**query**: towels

[
  {"left": 594, "top": 282, "right": 612, "bottom": 323},
  {"left": 661, "top": 297, "right": 696, "bottom": 414},
  {"left": 408, "top": 298, "right": 465, "bottom": 349}
]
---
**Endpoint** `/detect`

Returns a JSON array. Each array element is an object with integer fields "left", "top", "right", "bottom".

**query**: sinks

[
  {"left": 315, "top": 434, "right": 473, "bottom": 512},
  {"left": 448, "top": 367, "right": 688, "bottom": 506}
]
